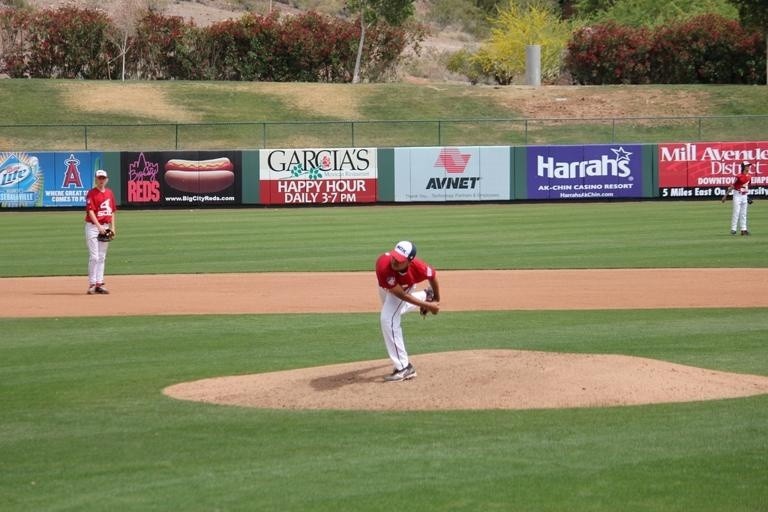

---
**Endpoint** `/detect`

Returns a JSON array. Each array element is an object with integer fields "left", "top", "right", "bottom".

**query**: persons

[
  {"left": 85, "top": 169, "right": 117, "bottom": 294},
  {"left": 376, "top": 240, "right": 441, "bottom": 382},
  {"left": 721, "top": 161, "right": 752, "bottom": 236}
]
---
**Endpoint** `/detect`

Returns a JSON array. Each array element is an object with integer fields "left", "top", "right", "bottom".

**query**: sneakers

[
  {"left": 85, "top": 283, "right": 110, "bottom": 295},
  {"left": 729, "top": 230, "right": 736, "bottom": 234},
  {"left": 740, "top": 229, "right": 749, "bottom": 235},
  {"left": 381, "top": 363, "right": 418, "bottom": 383}
]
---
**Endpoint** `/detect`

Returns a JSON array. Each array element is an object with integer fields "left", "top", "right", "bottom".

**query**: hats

[
  {"left": 740, "top": 160, "right": 751, "bottom": 167},
  {"left": 94, "top": 169, "right": 110, "bottom": 178},
  {"left": 388, "top": 239, "right": 417, "bottom": 263}
]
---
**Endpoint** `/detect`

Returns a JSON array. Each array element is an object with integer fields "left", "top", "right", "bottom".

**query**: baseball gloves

[
  {"left": 99, "top": 230, "right": 114, "bottom": 243},
  {"left": 747, "top": 198, "right": 753, "bottom": 204}
]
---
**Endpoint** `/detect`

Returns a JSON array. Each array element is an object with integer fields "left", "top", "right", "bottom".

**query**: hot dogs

[{"left": 164, "top": 156, "right": 235, "bottom": 193}]
